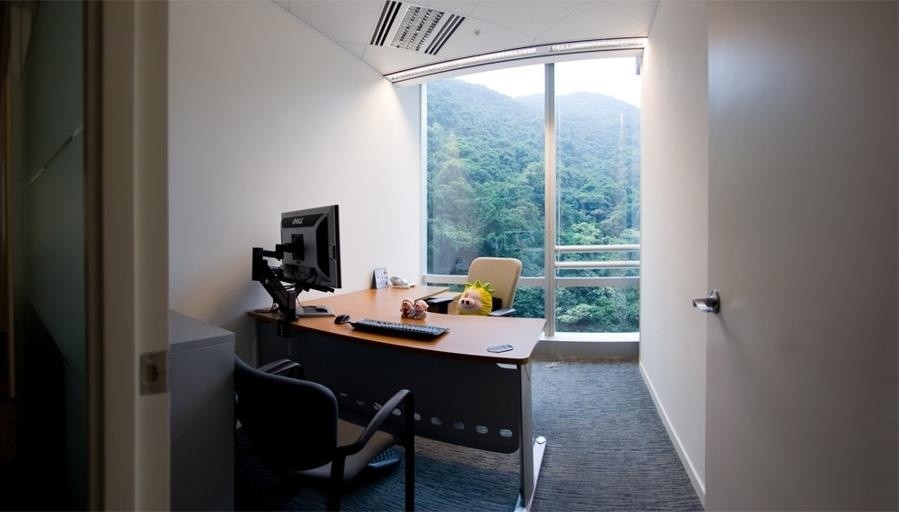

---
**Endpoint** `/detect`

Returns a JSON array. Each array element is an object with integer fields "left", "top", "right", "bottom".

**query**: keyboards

[{"left": 351, "top": 319, "right": 448, "bottom": 338}]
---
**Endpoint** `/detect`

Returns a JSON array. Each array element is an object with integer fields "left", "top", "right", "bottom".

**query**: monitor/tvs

[{"left": 252, "top": 205, "right": 341, "bottom": 323}]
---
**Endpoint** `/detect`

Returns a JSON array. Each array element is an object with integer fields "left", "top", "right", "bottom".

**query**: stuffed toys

[
  {"left": 400, "top": 298, "right": 429, "bottom": 320},
  {"left": 456, "top": 280, "right": 495, "bottom": 316}
]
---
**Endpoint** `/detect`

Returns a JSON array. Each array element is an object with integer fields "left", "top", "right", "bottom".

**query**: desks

[{"left": 247, "top": 285, "right": 547, "bottom": 512}]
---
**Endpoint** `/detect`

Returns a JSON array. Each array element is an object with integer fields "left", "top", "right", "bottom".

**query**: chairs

[
  {"left": 427, "top": 257, "right": 523, "bottom": 317},
  {"left": 235, "top": 355, "right": 415, "bottom": 512}
]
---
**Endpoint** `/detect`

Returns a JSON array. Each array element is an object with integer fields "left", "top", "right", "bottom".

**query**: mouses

[{"left": 335, "top": 314, "right": 349, "bottom": 324}]
